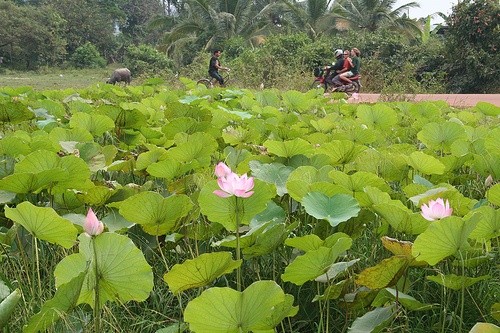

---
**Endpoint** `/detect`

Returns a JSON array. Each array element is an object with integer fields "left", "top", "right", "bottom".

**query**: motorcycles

[{"left": 312, "top": 62, "right": 361, "bottom": 93}]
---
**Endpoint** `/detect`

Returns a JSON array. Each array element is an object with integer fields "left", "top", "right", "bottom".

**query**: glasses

[{"left": 344, "top": 53, "right": 347, "bottom": 55}]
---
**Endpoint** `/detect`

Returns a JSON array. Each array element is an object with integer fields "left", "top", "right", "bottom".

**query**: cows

[{"left": 105, "top": 67, "right": 132, "bottom": 87}]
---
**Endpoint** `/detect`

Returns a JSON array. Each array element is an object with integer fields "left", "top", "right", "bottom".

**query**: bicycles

[{"left": 196, "top": 68, "right": 241, "bottom": 89}]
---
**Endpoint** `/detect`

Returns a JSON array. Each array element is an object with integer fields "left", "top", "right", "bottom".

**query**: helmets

[{"left": 334, "top": 49, "right": 343, "bottom": 58}]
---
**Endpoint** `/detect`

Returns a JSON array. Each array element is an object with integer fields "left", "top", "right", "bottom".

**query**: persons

[
  {"left": 326, "top": 48, "right": 360, "bottom": 91},
  {"left": 209, "top": 50, "right": 227, "bottom": 85}
]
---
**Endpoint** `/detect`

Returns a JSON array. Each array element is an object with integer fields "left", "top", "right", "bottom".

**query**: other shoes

[
  {"left": 339, "top": 85, "right": 346, "bottom": 89},
  {"left": 332, "top": 87, "right": 337, "bottom": 92},
  {"left": 348, "top": 81, "right": 354, "bottom": 88}
]
